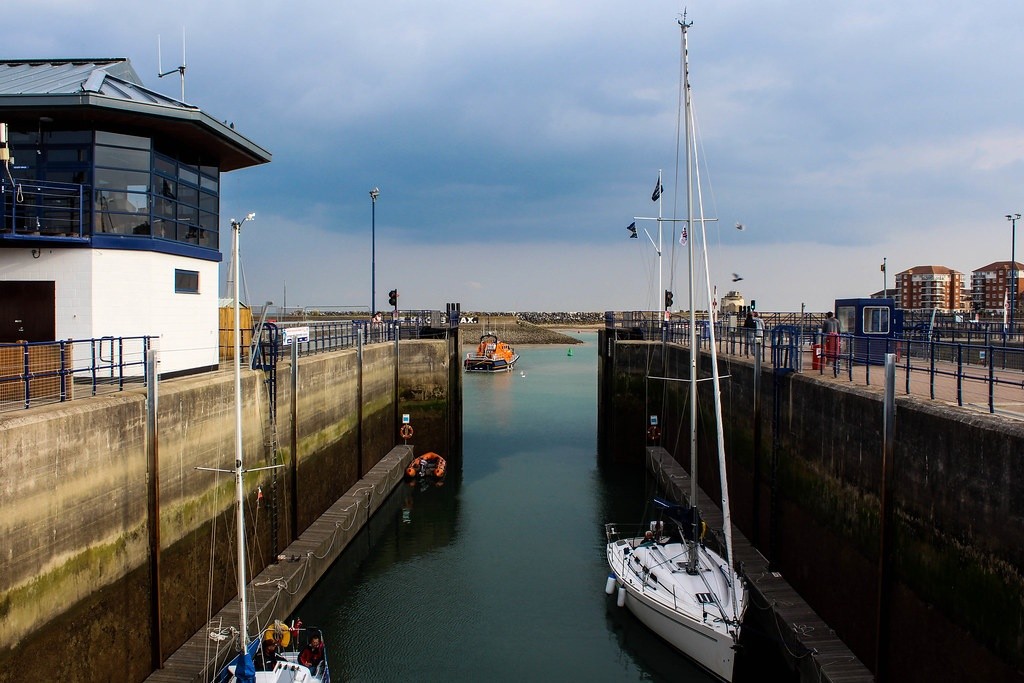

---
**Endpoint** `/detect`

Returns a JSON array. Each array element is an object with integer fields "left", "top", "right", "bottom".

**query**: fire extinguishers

[{"left": 291, "top": 616, "right": 302, "bottom": 637}]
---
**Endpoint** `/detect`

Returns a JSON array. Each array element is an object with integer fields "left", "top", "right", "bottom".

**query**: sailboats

[
  {"left": 198, "top": 215, "right": 332, "bottom": 683},
  {"left": 605, "top": 1, "right": 751, "bottom": 683}
]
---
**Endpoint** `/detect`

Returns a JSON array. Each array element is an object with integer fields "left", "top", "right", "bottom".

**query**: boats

[
  {"left": 464, "top": 315, "right": 520, "bottom": 373},
  {"left": 1005, "top": 214, "right": 1021, "bottom": 339},
  {"left": 405, "top": 452, "right": 446, "bottom": 480}
]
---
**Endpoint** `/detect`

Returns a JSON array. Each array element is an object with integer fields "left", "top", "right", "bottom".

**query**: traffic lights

[
  {"left": 751, "top": 300, "right": 755, "bottom": 309},
  {"left": 746, "top": 306, "right": 750, "bottom": 318},
  {"left": 739, "top": 306, "right": 743, "bottom": 318},
  {"left": 665, "top": 290, "right": 673, "bottom": 307},
  {"left": 389, "top": 290, "right": 396, "bottom": 306},
  {"left": 743, "top": 306, "right": 746, "bottom": 318}
]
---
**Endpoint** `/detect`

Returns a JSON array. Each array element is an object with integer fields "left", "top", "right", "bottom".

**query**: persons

[
  {"left": 371, "top": 312, "right": 382, "bottom": 343},
  {"left": 254, "top": 639, "right": 276, "bottom": 671},
  {"left": 640, "top": 531, "right": 655, "bottom": 545},
  {"left": 742, "top": 313, "right": 755, "bottom": 355},
  {"left": 822, "top": 311, "right": 841, "bottom": 367},
  {"left": 753, "top": 312, "right": 765, "bottom": 356},
  {"left": 298, "top": 633, "right": 325, "bottom": 675}
]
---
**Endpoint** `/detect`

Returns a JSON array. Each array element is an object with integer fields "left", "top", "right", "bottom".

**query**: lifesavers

[
  {"left": 647, "top": 426, "right": 661, "bottom": 440},
  {"left": 265, "top": 623, "right": 291, "bottom": 648},
  {"left": 400, "top": 424, "right": 414, "bottom": 439},
  {"left": 699, "top": 520, "right": 706, "bottom": 539}
]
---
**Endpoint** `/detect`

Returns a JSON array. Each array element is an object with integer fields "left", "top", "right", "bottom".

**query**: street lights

[{"left": 369, "top": 187, "right": 379, "bottom": 318}]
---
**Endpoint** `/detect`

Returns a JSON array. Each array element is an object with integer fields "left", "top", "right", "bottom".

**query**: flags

[
  {"left": 652, "top": 176, "right": 664, "bottom": 202},
  {"left": 679, "top": 223, "right": 687, "bottom": 247},
  {"left": 626, "top": 222, "right": 637, "bottom": 239}
]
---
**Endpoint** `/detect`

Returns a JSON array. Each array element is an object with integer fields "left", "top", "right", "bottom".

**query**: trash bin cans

[{"left": 812, "top": 344, "right": 826, "bottom": 370}]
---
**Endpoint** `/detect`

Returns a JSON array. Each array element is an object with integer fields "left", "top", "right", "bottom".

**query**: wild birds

[
  {"left": 222, "top": 120, "right": 235, "bottom": 130},
  {"left": 732, "top": 273, "right": 744, "bottom": 282},
  {"left": 736, "top": 223, "right": 743, "bottom": 230}
]
